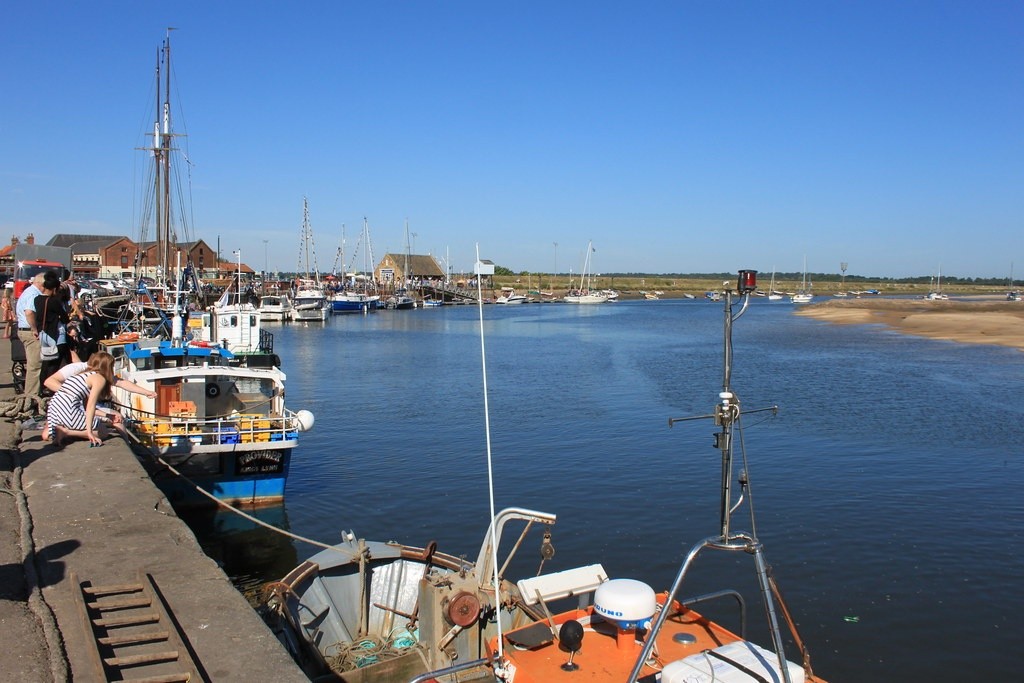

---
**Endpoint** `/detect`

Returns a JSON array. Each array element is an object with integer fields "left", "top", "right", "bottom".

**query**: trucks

[{"left": 11, "top": 243, "right": 75, "bottom": 320}]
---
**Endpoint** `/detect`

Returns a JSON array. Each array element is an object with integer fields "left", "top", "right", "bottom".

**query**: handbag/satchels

[{"left": 39, "top": 330, "right": 59, "bottom": 360}]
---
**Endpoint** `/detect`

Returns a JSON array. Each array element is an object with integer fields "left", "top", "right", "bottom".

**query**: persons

[
  {"left": 14, "top": 270, "right": 107, "bottom": 409},
  {"left": 0, "top": 288, "right": 14, "bottom": 340},
  {"left": 41, "top": 350, "right": 158, "bottom": 447}
]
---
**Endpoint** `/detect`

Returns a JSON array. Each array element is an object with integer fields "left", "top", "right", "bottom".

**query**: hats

[{"left": 43, "top": 271, "right": 61, "bottom": 290}]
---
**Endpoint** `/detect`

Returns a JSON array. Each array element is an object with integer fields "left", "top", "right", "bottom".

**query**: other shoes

[
  {"left": 22, "top": 410, "right": 43, "bottom": 423},
  {"left": 2, "top": 335, "right": 10, "bottom": 339}
]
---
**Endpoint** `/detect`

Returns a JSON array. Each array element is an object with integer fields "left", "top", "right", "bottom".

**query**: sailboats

[{"left": 48, "top": 23, "right": 1024, "bottom": 511}]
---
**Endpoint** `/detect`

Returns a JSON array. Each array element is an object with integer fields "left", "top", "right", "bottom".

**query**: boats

[{"left": 262, "top": 239, "right": 829, "bottom": 683}]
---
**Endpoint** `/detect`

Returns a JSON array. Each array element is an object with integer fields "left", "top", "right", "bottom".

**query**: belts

[{"left": 18, "top": 328, "right": 31, "bottom": 331}]
